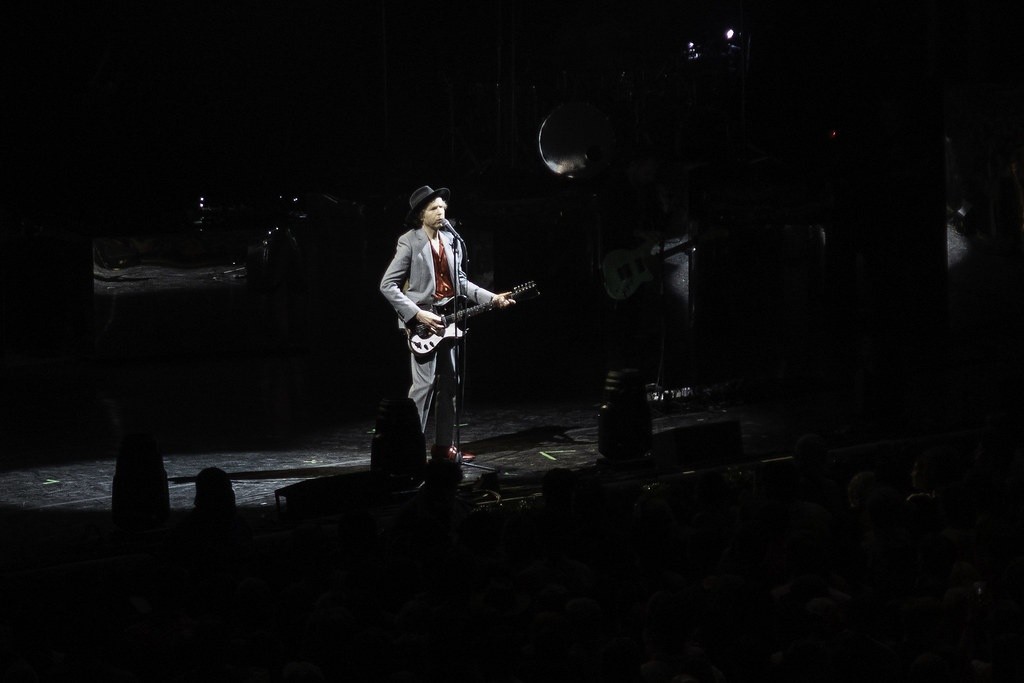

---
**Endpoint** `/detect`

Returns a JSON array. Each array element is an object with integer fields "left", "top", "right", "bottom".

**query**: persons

[
  {"left": 379, "top": 186, "right": 516, "bottom": 462},
  {"left": 602, "top": 156, "right": 659, "bottom": 379},
  {"left": 0, "top": 413, "right": 1024, "bottom": 683}
]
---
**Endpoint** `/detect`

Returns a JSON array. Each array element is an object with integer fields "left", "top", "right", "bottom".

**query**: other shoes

[{"left": 431, "top": 443, "right": 476, "bottom": 462}]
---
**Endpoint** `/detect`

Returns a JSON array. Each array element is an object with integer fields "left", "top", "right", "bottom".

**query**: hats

[{"left": 409, "top": 185, "right": 452, "bottom": 219}]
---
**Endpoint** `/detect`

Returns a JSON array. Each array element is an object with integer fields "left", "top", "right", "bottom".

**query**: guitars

[
  {"left": 395, "top": 279, "right": 542, "bottom": 366},
  {"left": 599, "top": 226, "right": 731, "bottom": 303}
]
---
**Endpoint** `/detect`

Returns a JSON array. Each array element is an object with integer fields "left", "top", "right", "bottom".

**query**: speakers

[
  {"left": 648, "top": 418, "right": 752, "bottom": 475},
  {"left": 275, "top": 468, "right": 378, "bottom": 518}
]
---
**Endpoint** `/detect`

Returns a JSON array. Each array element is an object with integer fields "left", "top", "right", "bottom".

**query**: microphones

[{"left": 441, "top": 218, "right": 465, "bottom": 243}]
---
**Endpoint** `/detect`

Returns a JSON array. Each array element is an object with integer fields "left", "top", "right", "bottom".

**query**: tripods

[{"left": 447, "top": 235, "right": 500, "bottom": 473}]
